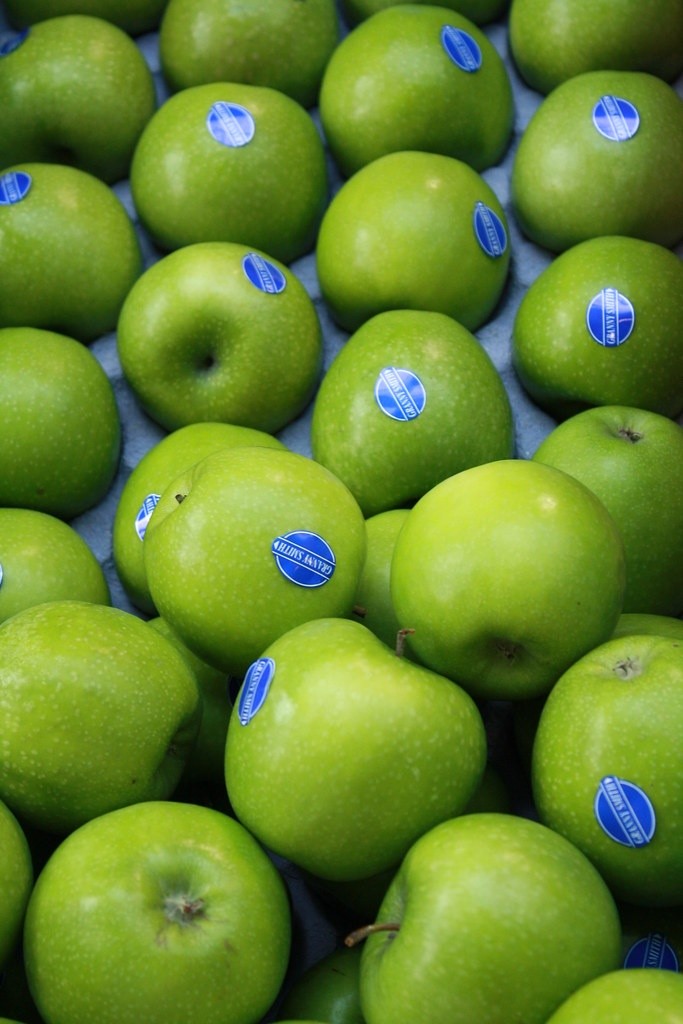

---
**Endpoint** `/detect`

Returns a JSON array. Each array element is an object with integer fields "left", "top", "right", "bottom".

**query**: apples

[{"left": 0, "top": 0, "right": 683, "bottom": 1024}]
